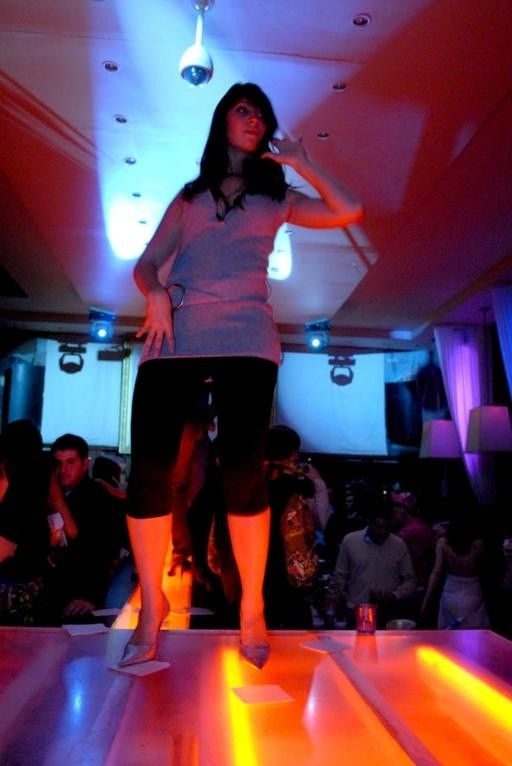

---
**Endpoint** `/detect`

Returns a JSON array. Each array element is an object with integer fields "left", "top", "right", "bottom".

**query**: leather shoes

[
  {"left": 239, "top": 601, "right": 272, "bottom": 670},
  {"left": 116, "top": 589, "right": 172, "bottom": 670}
]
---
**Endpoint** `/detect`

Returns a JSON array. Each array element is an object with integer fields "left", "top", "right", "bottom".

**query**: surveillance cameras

[{"left": 180, "top": 45, "right": 213, "bottom": 86}]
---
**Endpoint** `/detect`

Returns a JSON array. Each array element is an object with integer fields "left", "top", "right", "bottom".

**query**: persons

[
  {"left": 118, "top": 83, "right": 365, "bottom": 670},
  {"left": 0, "top": 418, "right": 512, "bottom": 630}
]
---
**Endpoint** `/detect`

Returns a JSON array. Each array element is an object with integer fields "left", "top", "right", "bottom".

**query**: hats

[{"left": 389, "top": 489, "right": 418, "bottom": 510}]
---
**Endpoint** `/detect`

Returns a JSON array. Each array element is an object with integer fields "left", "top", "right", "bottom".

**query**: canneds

[{"left": 356, "top": 603, "right": 376, "bottom": 634}]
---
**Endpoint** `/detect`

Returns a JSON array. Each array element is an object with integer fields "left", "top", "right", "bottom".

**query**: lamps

[
  {"left": 87, "top": 306, "right": 117, "bottom": 341},
  {"left": 302, "top": 315, "right": 332, "bottom": 353},
  {"left": 327, "top": 353, "right": 358, "bottom": 384},
  {"left": 55, "top": 341, "right": 86, "bottom": 373},
  {"left": 466, "top": 404, "right": 512, "bottom": 463},
  {"left": 418, "top": 417, "right": 464, "bottom": 457}
]
